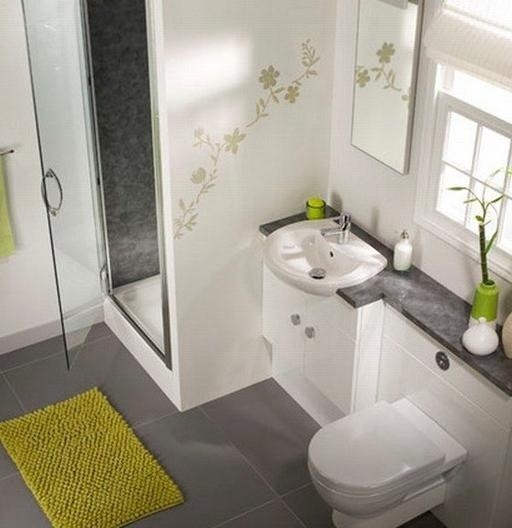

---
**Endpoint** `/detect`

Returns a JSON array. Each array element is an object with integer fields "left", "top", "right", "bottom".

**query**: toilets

[{"left": 307, "top": 395, "right": 467, "bottom": 525}]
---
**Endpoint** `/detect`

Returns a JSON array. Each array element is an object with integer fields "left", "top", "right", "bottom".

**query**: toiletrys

[{"left": 394, "top": 228, "right": 413, "bottom": 272}]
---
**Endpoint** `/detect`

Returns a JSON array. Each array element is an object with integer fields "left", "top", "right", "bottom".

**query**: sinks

[{"left": 265, "top": 216, "right": 387, "bottom": 292}]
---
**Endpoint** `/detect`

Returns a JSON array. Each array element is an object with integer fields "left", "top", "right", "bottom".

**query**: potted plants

[{"left": 446, "top": 168, "right": 512, "bottom": 331}]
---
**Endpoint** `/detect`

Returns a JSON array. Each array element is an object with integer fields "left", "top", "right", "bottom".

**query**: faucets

[{"left": 319, "top": 210, "right": 351, "bottom": 245}]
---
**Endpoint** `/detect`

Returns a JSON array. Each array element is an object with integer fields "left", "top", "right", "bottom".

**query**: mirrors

[{"left": 350, "top": 0, "right": 424, "bottom": 175}]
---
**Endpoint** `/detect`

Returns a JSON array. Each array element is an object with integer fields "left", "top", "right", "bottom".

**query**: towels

[{"left": 0, "top": 156, "right": 15, "bottom": 258}]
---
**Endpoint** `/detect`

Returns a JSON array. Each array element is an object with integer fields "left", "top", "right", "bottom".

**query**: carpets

[{"left": 0, "top": 387, "right": 185, "bottom": 528}]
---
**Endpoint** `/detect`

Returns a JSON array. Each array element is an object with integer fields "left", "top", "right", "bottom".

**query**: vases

[{"left": 462, "top": 317, "right": 499, "bottom": 356}]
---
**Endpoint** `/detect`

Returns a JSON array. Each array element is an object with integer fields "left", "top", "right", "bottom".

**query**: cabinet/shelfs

[{"left": 262, "top": 260, "right": 384, "bottom": 427}]
